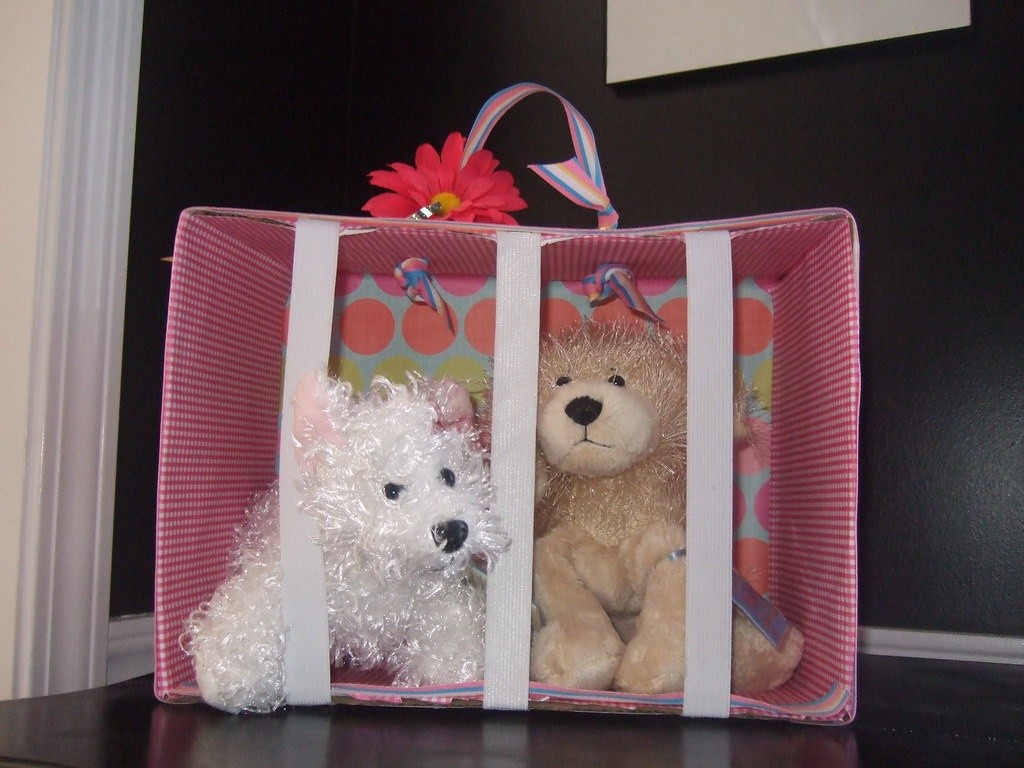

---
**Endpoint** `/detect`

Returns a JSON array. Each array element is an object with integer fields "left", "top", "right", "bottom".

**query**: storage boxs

[{"left": 159, "top": 206, "right": 861, "bottom": 727}]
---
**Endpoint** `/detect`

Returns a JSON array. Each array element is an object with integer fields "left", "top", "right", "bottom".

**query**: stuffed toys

[
  {"left": 177, "top": 371, "right": 511, "bottom": 714},
  {"left": 476, "top": 317, "right": 804, "bottom": 691}
]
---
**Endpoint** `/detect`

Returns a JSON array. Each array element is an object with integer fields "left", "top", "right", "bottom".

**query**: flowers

[{"left": 362, "top": 133, "right": 530, "bottom": 225}]
